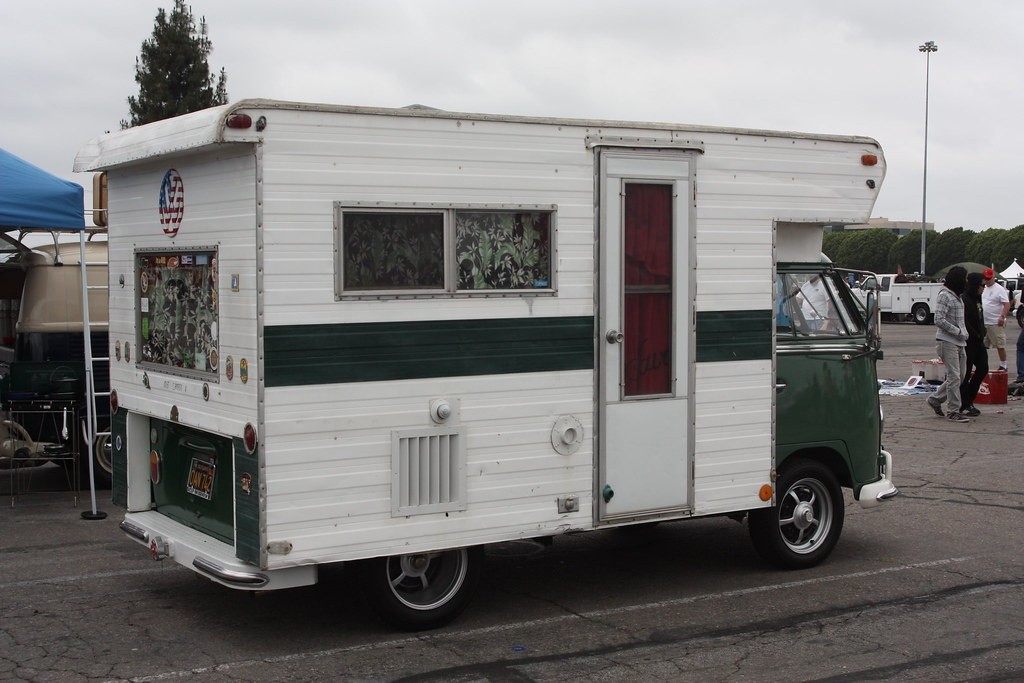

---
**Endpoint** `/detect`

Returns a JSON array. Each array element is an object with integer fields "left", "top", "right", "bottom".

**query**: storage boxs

[
  {"left": 939, "top": 362, "right": 950, "bottom": 382},
  {"left": 974, "top": 371, "right": 1009, "bottom": 405},
  {"left": 911, "top": 360, "right": 937, "bottom": 383}
]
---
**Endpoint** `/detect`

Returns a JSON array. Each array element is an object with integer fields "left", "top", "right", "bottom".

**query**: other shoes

[{"left": 998, "top": 366, "right": 1008, "bottom": 371}]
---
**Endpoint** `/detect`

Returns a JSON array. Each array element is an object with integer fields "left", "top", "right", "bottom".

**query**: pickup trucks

[
  {"left": 849, "top": 276, "right": 949, "bottom": 327},
  {"left": 997, "top": 279, "right": 1022, "bottom": 310}
]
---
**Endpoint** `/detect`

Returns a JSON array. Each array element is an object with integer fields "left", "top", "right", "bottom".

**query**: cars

[{"left": 0, "top": 421, "right": 55, "bottom": 467}]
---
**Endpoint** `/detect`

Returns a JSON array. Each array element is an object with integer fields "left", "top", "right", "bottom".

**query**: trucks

[
  {"left": 70, "top": 99, "right": 895, "bottom": 623},
  {"left": 0, "top": 242, "right": 108, "bottom": 485}
]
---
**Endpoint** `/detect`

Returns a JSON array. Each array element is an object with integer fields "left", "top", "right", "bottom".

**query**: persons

[
  {"left": 926, "top": 265, "right": 989, "bottom": 422},
  {"left": 981, "top": 268, "right": 1024, "bottom": 383},
  {"left": 795, "top": 274, "right": 843, "bottom": 331},
  {"left": 844, "top": 276, "right": 865, "bottom": 289}
]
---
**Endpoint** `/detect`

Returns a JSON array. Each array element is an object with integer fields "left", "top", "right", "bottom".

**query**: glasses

[{"left": 980, "top": 284, "right": 985, "bottom": 287}]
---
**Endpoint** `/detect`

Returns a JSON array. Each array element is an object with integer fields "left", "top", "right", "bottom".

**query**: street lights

[{"left": 919, "top": 41, "right": 940, "bottom": 275}]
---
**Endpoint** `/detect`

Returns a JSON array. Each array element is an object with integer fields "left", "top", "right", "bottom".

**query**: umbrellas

[{"left": 995, "top": 258, "right": 1024, "bottom": 278}]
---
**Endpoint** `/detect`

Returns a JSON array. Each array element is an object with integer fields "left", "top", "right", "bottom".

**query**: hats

[{"left": 983, "top": 269, "right": 993, "bottom": 278}]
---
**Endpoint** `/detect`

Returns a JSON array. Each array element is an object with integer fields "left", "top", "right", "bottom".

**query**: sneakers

[
  {"left": 947, "top": 412, "right": 970, "bottom": 422},
  {"left": 927, "top": 398, "right": 945, "bottom": 417},
  {"left": 960, "top": 406, "right": 981, "bottom": 416}
]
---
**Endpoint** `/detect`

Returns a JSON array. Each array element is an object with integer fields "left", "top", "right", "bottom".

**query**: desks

[{"left": 8, "top": 400, "right": 81, "bottom": 507}]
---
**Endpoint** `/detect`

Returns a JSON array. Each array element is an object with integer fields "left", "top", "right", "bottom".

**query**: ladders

[{"left": 80, "top": 228, "right": 113, "bottom": 521}]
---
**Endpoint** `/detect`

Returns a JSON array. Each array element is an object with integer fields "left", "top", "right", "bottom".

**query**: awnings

[{"left": 0, "top": 148, "right": 108, "bottom": 520}]
